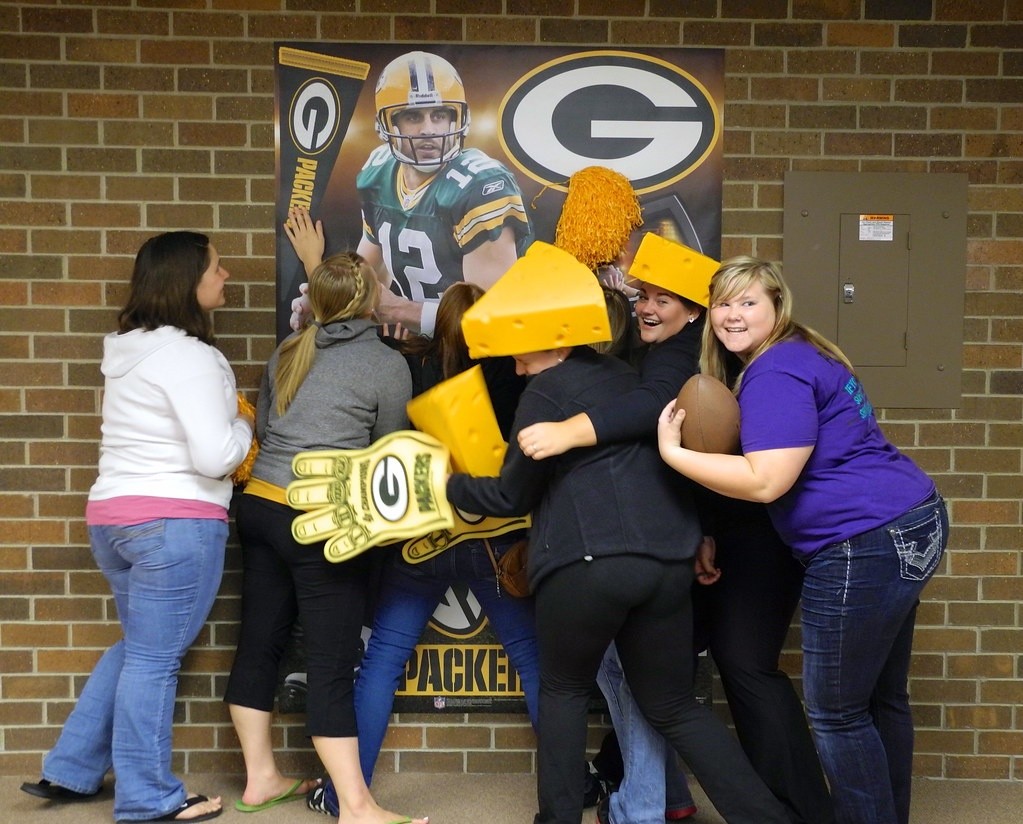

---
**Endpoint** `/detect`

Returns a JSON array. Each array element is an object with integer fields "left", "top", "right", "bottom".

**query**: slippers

[
  {"left": 235, "top": 780, "right": 317, "bottom": 812},
  {"left": 20, "top": 774, "right": 103, "bottom": 800},
  {"left": 389, "top": 815, "right": 411, "bottom": 824},
  {"left": 117, "top": 792, "right": 222, "bottom": 824}
]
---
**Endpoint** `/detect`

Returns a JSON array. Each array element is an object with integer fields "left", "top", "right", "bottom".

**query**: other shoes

[
  {"left": 585, "top": 780, "right": 610, "bottom": 809},
  {"left": 665, "top": 804, "right": 697, "bottom": 820},
  {"left": 595, "top": 796, "right": 611, "bottom": 824},
  {"left": 305, "top": 784, "right": 340, "bottom": 817}
]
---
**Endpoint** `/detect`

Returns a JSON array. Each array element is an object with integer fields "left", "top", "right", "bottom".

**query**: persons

[
  {"left": 289, "top": 51, "right": 532, "bottom": 342},
  {"left": 657, "top": 256, "right": 950, "bottom": 824},
  {"left": 442, "top": 276, "right": 834, "bottom": 824},
  {"left": 222, "top": 252, "right": 429, "bottom": 824},
  {"left": 276, "top": 206, "right": 604, "bottom": 822},
  {"left": 20, "top": 231, "right": 256, "bottom": 824}
]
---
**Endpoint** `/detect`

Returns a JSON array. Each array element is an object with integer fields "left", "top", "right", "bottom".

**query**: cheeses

[
  {"left": 460, "top": 241, "right": 615, "bottom": 359},
  {"left": 407, "top": 364, "right": 511, "bottom": 500},
  {"left": 627, "top": 231, "right": 720, "bottom": 309}
]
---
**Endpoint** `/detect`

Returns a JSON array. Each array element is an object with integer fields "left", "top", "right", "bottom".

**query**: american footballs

[{"left": 674, "top": 371, "right": 742, "bottom": 455}]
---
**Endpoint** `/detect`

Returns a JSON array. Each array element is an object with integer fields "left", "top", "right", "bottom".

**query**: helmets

[{"left": 373, "top": 51, "right": 469, "bottom": 172}]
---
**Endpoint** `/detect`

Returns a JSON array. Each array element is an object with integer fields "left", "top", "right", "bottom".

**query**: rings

[
  {"left": 697, "top": 572, "right": 705, "bottom": 578},
  {"left": 532, "top": 444, "right": 538, "bottom": 453}
]
